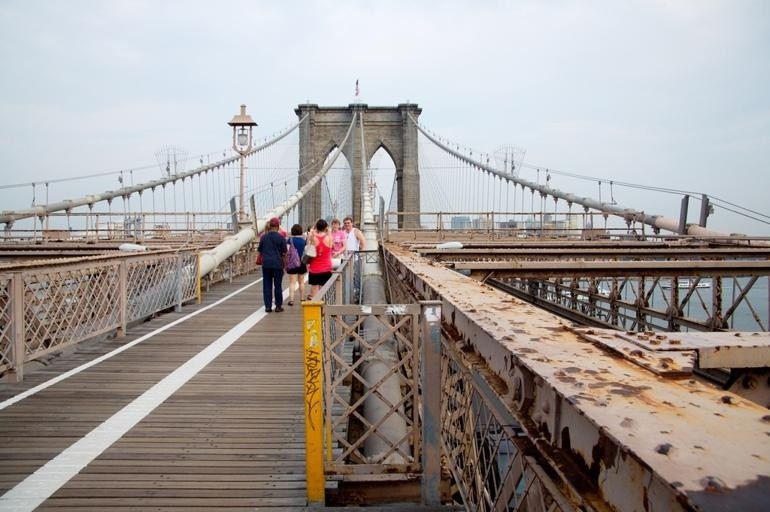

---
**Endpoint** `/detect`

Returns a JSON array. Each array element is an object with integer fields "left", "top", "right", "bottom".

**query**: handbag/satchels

[
  {"left": 286, "top": 246, "right": 300, "bottom": 271},
  {"left": 301, "top": 245, "right": 317, "bottom": 263},
  {"left": 331, "top": 258, "right": 341, "bottom": 270},
  {"left": 256, "top": 255, "right": 263, "bottom": 265}
]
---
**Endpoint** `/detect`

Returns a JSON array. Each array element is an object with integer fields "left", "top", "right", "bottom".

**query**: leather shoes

[
  {"left": 266, "top": 307, "right": 272, "bottom": 312},
  {"left": 275, "top": 307, "right": 283, "bottom": 311}
]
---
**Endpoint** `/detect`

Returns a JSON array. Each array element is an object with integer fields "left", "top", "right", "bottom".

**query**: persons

[{"left": 256, "top": 215, "right": 368, "bottom": 313}]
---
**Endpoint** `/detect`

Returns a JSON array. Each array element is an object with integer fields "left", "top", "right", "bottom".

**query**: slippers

[
  {"left": 307, "top": 295, "right": 313, "bottom": 300},
  {"left": 288, "top": 301, "right": 294, "bottom": 305},
  {"left": 301, "top": 300, "right": 305, "bottom": 301}
]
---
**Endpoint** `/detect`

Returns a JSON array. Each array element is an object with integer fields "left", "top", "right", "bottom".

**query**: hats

[{"left": 270, "top": 218, "right": 279, "bottom": 226}]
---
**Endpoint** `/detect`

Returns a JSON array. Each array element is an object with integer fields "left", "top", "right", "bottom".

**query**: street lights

[
  {"left": 227, "top": 104, "right": 258, "bottom": 221},
  {"left": 116, "top": 242, "right": 172, "bottom": 254},
  {"left": 402, "top": 240, "right": 464, "bottom": 251}
]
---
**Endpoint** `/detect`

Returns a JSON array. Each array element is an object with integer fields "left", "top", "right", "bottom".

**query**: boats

[
  {"left": 660, "top": 279, "right": 710, "bottom": 291},
  {"left": 548, "top": 286, "right": 614, "bottom": 303}
]
